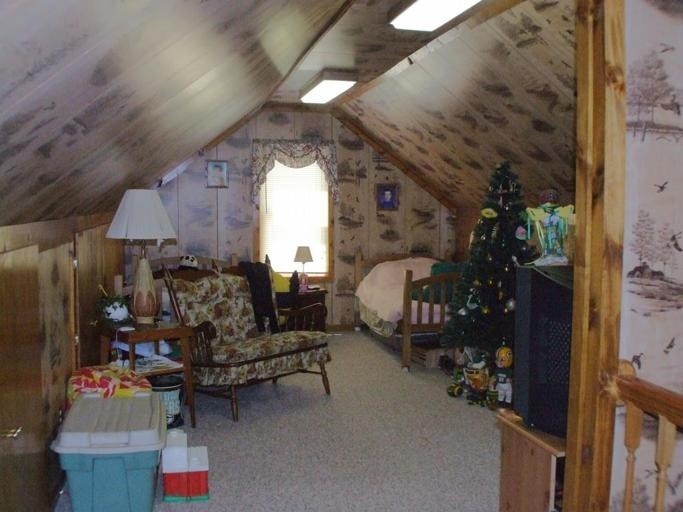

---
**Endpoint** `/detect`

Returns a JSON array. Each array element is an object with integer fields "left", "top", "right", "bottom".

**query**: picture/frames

[
  {"left": 374, "top": 182, "right": 399, "bottom": 211},
  {"left": 205, "top": 160, "right": 228, "bottom": 188}
]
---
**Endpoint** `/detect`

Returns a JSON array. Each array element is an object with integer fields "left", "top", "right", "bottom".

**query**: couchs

[{"left": 162, "top": 255, "right": 332, "bottom": 421}]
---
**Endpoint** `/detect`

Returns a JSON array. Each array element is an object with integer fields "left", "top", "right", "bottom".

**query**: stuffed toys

[
  {"left": 177, "top": 253, "right": 200, "bottom": 270},
  {"left": 490, "top": 345, "right": 513, "bottom": 407}
]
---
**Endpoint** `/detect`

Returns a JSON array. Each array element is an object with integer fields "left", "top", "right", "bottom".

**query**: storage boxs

[
  {"left": 410, "top": 333, "right": 444, "bottom": 369},
  {"left": 160, "top": 445, "right": 211, "bottom": 503},
  {"left": 50, "top": 389, "right": 167, "bottom": 512}
]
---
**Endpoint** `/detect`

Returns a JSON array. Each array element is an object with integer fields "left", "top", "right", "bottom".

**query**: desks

[
  {"left": 274, "top": 286, "right": 328, "bottom": 333},
  {"left": 497, "top": 408, "right": 566, "bottom": 512},
  {"left": 97, "top": 315, "right": 198, "bottom": 429}
]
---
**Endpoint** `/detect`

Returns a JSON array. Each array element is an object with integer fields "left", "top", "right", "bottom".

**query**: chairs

[{"left": 225, "top": 263, "right": 273, "bottom": 277}]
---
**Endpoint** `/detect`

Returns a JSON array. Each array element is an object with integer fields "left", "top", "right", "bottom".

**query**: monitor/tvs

[{"left": 513, "top": 264, "right": 574, "bottom": 440}]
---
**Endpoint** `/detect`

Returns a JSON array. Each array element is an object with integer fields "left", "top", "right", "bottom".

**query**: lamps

[
  {"left": 296, "top": 67, "right": 358, "bottom": 104},
  {"left": 104, "top": 190, "right": 177, "bottom": 325},
  {"left": 387, "top": 1, "right": 481, "bottom": 32},
  {"left": 295, "top": 246, "right": 312, "bottom": 294}
]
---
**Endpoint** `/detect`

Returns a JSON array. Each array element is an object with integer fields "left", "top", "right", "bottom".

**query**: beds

[
  {"left": 351, "top": 245, "right": 463, "bottom": 372},
  {"left": 114, "top": 253, "right": 239, "bottom": 320}
]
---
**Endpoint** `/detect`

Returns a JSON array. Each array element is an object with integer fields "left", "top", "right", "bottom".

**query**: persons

[{"left": 380, "top": 189, "right": 396, "bottom": 208}]
